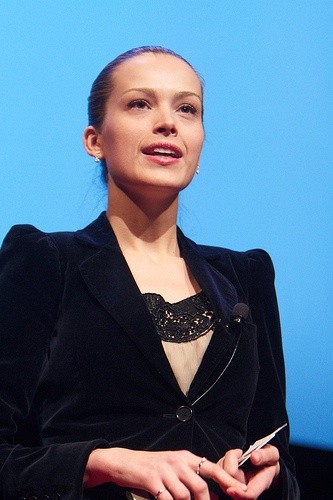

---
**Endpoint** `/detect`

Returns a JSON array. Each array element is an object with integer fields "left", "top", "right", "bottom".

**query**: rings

[
  {"left": 197, "top": 457, "right": 206, "bottom": 475},
  {"left": 155, "top": 488, "right": 166, "bottom": 498}
]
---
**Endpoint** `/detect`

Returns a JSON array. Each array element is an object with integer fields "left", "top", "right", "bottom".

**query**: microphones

[{"left": 186, "top": 302, "right": 250, "bottom": 408}]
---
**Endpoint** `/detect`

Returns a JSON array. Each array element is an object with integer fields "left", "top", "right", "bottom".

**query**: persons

[{"left": 0, "top": 45, "right": 300, "bottom": 500}]
